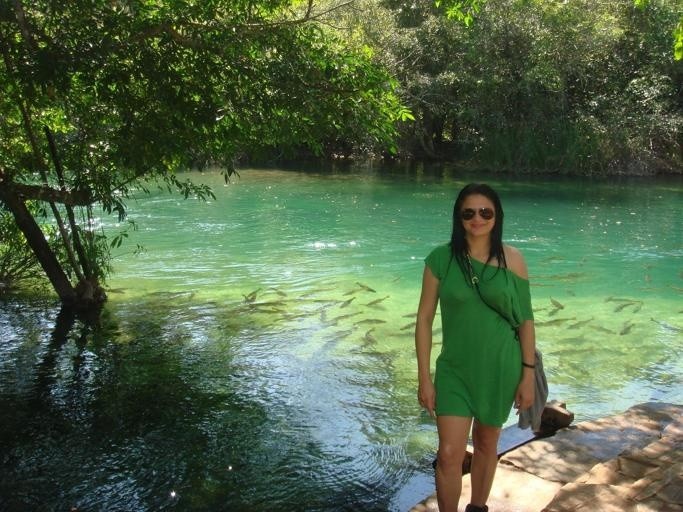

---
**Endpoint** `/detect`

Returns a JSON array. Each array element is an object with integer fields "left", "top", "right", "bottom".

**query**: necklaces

[{"left": 466, "top": 251, "right": 487, "bottom": 285}]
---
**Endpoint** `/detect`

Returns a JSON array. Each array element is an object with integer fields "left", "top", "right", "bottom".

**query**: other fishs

[{"left": 101, "top": 237, "right": 682, "bottom": 393}]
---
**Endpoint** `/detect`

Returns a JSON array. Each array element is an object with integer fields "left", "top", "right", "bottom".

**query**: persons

[{"left": 413, "top": 182, "right": 538, "bottom": 512}]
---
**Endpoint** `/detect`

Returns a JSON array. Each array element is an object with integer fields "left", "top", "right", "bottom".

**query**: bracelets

[{"left": 523, "top": 362, "right": 535, "bottom": 367}]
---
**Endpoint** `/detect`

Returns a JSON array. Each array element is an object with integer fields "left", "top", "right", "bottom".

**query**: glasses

[{"left": 461, "top": 208, "right": 494, "bottom": 220}]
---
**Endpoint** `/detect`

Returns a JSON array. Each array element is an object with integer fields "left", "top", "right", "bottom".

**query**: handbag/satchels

[{"left": 518, "top": 348, "right": 547, "bottom": 432}]
